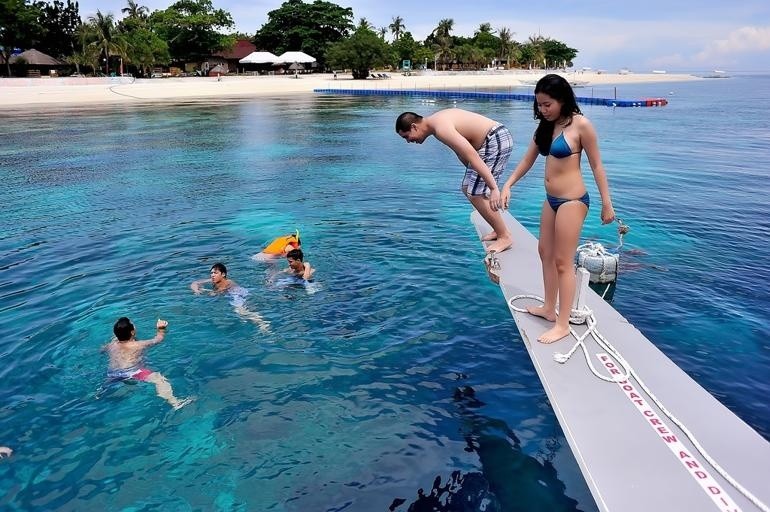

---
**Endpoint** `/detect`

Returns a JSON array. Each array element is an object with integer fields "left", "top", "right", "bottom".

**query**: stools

[{"left": 246, "top": 70, "right": 274, "bottom": 75}]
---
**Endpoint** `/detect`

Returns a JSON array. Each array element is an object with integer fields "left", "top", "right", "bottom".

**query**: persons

[
  {"left": 393, "top": 109, "right": 514, "bottom": 255},
  {"left": 498, "top": 75, "right": 615, "bottom": 342},
  {"left": 267, "top": 250, "right": 316, "bottom": 292},
  {"left": 0, "top": 446, "right": 15, "bottom": 461},
  {"left": 97, "top": 318, "right": 198, "bottom": 410},
  {"left": 191, "top": 264, "right": 276, "bottom": 339}
]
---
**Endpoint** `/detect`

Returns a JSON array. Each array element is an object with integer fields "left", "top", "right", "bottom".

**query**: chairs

[
  {"left": 371, "top": 73, "right": 391, "bottom": 79},
  {"left": 150, "top": 67, "right": 182, "bottom": 77},
  {"left": 28, "top": 69, "right": 58, "bottom": 78}
]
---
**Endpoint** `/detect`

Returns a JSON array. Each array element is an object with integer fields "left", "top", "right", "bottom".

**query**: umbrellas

[
  {"left": 210, "top": 65, "right": 227, "bottom": 83},
  {"left": 273, "top": 52, "right": 319, "bottom": 62},
  {"left": 239, "top": 52, "right": 279, "bottom": 65},
  {"left": 289, "top": 63, "right": 306, "bottom": 79}
]
